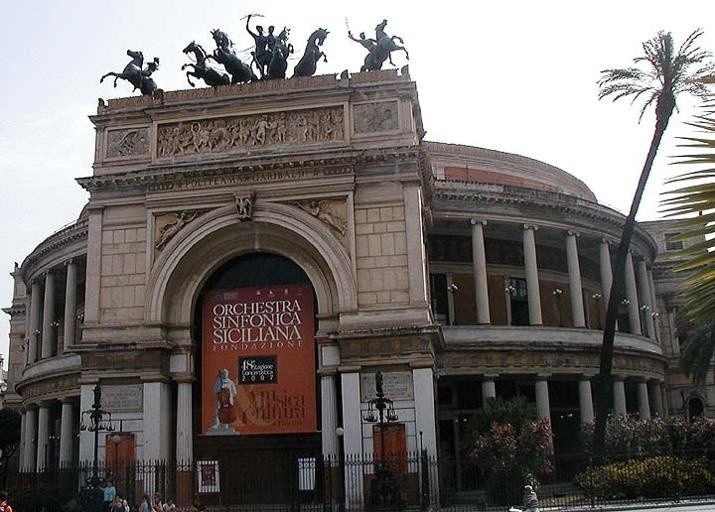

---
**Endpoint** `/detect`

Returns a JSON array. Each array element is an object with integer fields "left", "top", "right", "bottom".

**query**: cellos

[{"left": 216, "top": 370, "right": 237, "bottom": 424}]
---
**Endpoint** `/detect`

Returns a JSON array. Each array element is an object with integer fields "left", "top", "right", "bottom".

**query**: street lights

[
  {"left": 652, "top": 311, "right": 660, "bottom": 344},
  {"left": 593, "top": 292, "right": 601, "bottom": 330},
  {"left": 77, "top": 312, "right": 84, "bottom": 340},
  {"left": 50, "top": 321, "right": 60, "bottom": 356},
  {"left": 80, "top": 383, "right": 115, "bottom": 511},
  {"left": 553, "top": 287, "right": 564, "bottom": 327},
  {"left": 364, "top": 370, "right": 399, "bottom": 512},
  {"left": 33, "top": 329, "right": 41, "bottom": 362},
  {"left": 504, "top": 284, "right": 516, "bottom": 325},
  {"left": 448, "top": 283, "right": 459, "bottom": 325},
  {"left": 641, "top": 304, "right": 649, "bottom": 338},
  {"left": 621, "top": 298, "right": 630, "bottom": 332},
  {"left": 24, "top": 337, "right": 31, "bottom": 366}
]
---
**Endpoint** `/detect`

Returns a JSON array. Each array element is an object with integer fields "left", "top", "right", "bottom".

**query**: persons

[
  {"left": 0, "top": 490, "right": 12, "bottom": 511},
  {"left": 156, "top": 211, "right": 197, "bottom": 247},
  {"left": 108, "top": 491, "right": 177, "bottom": 512},
  {"left": 209, "top": 367, "right": 237, "bottom": 429},
  {"left": 263, "top": 25, "right": 280, "bottom": 51},
  {"left": 157, "top": 109, "right": 334, "bottom": 155},
  {"left": 248, "top": 14, "right": 268, "bottom": 82},
  {"left": 347, "top": 30, "right": 378, "bottom": 52},
  {"left": 294, "top": 199, "right": 347, "bottom": 236},
  {"left": 519, "top": 483, "right": 539, "bottom": 511},
  {"left": 141, "top": 57, "right": 160, "bottom": 78},
  {"left": 98, "top": 480, "right": 117, "bottom": 512}
]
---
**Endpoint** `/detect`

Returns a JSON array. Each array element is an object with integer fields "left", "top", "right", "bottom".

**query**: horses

[
  {"left": 263, "top": 25, "right": 294, "bottom": 80},
  {"left": 180, "top": 40, "right": 230, "bottom": 88},
  {"left": 359, "top": 18, "right": 409, "bottom": 72},
  {"left": 99, "top": 48, "right": 158, "bottom": 96},
  {"left": 202, "top": 28, "right": 260, "bottom": 85},
  {"left": 290, "top": 26, "right": 331, "bottom": 80}
]
